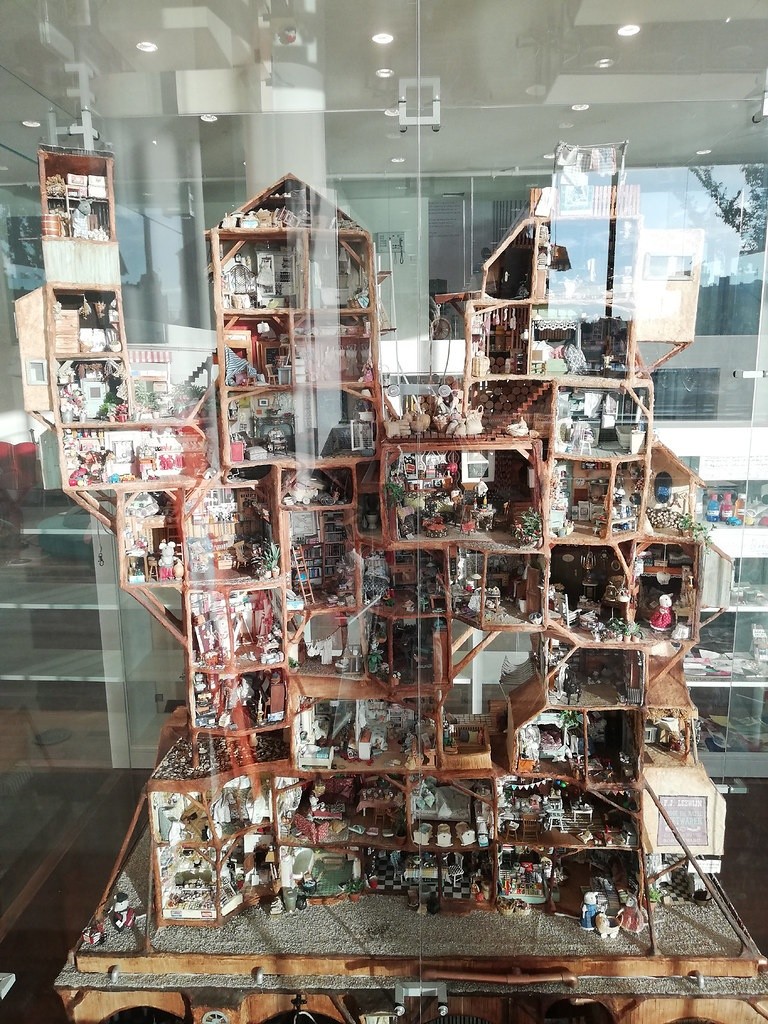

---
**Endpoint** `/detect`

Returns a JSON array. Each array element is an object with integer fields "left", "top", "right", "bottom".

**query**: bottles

[{"left": 706, "top": 493, "right": 745, "bottom": 522}]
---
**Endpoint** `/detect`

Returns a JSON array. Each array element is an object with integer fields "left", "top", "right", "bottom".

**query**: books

[{"left": 679, "top": 626, "right": 768, "bottom": 679}]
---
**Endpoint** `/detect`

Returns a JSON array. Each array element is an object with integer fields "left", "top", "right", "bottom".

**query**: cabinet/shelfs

[
  {"left": 0, "top": 507, "right": 184, "bottom": 684},
  {"left": 90, "top": 427, "right": 768, "bottom": 779}
]
